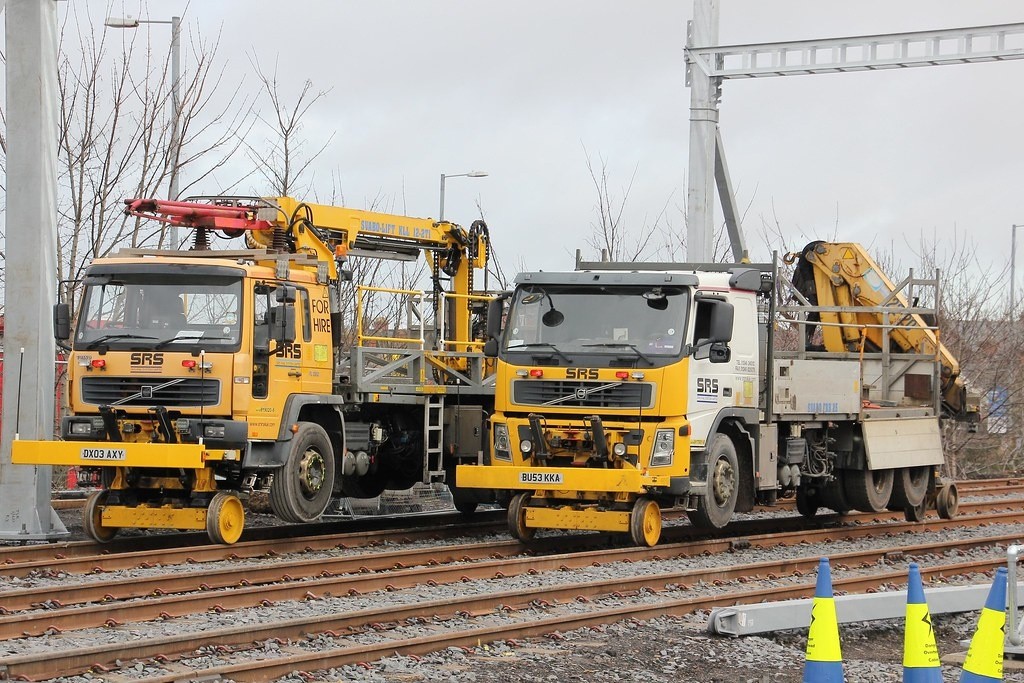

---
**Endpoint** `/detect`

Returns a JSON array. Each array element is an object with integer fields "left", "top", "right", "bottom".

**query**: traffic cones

[
  {"left": 802, "top": 557, "right": 845, "bottom": 683},
  {"left": 960, "top": 567, "right": 1008, "bottom": 683},
  {"left": 902, "top": 563, "right": 944, "bottom": 683}
]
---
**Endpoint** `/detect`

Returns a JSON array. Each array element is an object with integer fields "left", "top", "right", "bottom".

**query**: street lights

[
  {"left": 439, "top": 171, "right": 488, "bottom": 222},
  {"left": 104, "top": 16, "right": 180, "bottom": 252}
]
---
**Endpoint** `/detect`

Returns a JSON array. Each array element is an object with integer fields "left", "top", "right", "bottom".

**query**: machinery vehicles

[
  {"left": 457, "top": 240, "right": 982, "bottom": 547},
  {"left": 12, "top": 195, "right": 516, "bottom": 545}
]
